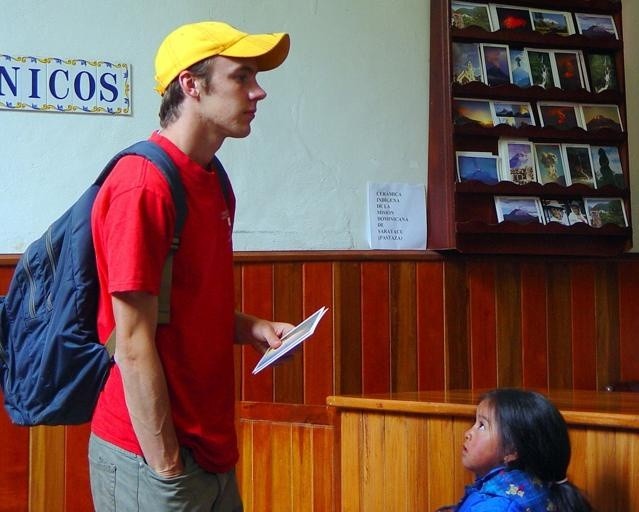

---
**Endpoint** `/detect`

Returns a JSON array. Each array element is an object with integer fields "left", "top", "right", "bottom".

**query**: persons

[
  {"left": 568, "top": 200, "right": 588, "bottom": 226},
  {"left": 433, "top": 388, "right": 594, "bottom": 511},
  {"left": 0, "top": 20, "right": 302, "bottom": 511},
  {"left": 545, "top": 200, "right": 569, "bottom": 226}
]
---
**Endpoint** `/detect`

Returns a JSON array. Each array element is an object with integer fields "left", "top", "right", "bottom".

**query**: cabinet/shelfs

[{"left": 425, "top": 0, "right": 635, "bottom": 256}]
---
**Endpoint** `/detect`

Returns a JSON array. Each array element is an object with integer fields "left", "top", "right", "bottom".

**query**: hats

[{"left": 153, "top": 21, "right": 289, "bottom": 95}]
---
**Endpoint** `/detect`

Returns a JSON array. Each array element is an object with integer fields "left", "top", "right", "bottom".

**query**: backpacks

[{"left": 0, "top": 141, "right": 232, "bottom": 426}]
